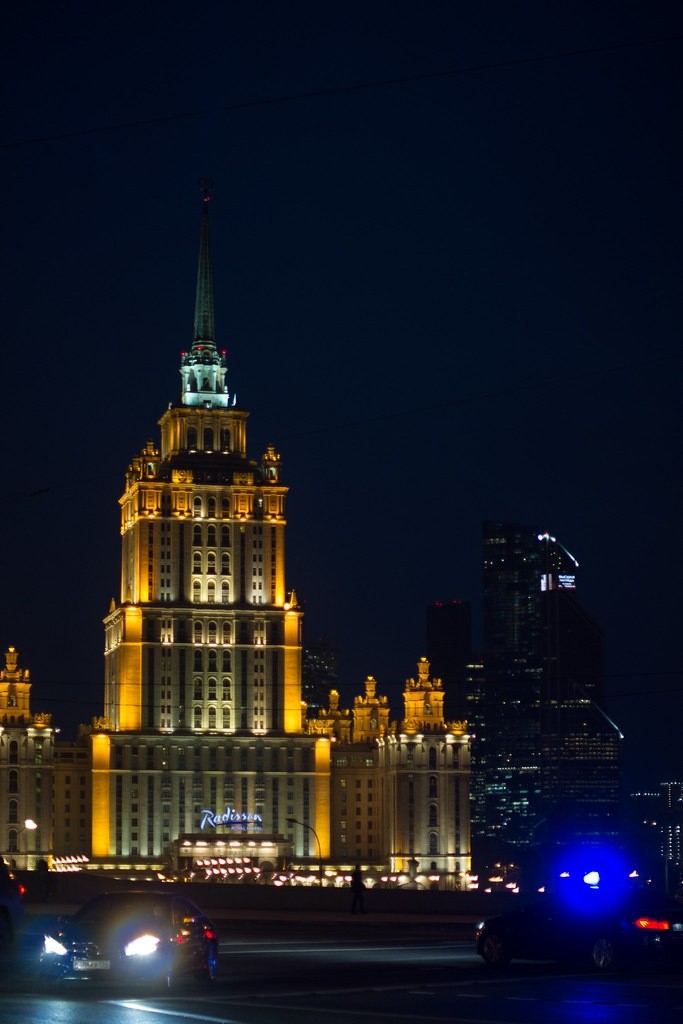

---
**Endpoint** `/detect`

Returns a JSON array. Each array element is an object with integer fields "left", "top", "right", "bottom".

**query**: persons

[
  {"left": 36, "top": 860, "right": 49, "bottom": 897},
  {"left": 351, "top": 864, "right": 368, "bottom": 915}
]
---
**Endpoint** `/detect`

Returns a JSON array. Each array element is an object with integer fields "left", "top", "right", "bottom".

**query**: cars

[
  {"left": 34, "top": 888, "right": 219, "bottom": 999},
  {"left": 0, "top": 855, "right": 27, "bottom": 952},
  {"left": 473, "top": 908, "right": 653, "bottom": 977}
]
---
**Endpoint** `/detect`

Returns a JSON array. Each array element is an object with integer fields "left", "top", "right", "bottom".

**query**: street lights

[{"left": 284, "top": 814, "right": 325, "bottom": 886}]
